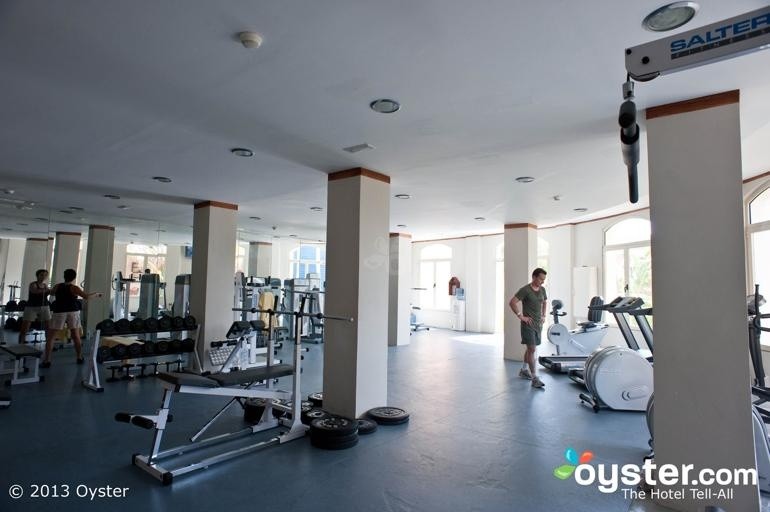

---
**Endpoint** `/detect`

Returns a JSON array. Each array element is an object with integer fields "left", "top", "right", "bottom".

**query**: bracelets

[
  {"left": 542, "top": 315, "right": 546, "bottom": 317},
  {"left": 517, "top": 312, "right": 521, "bottom": 316}
]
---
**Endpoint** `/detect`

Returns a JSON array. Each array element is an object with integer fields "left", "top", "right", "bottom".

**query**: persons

[
  {"left": 18, "top": 270, "right": 54, "bottom": 345},
  {"left": 38, "top": 269, "right": 104, "bottom": 367},
  {"left": 509, "top": 268, "right": 548, "bottom": 388}
]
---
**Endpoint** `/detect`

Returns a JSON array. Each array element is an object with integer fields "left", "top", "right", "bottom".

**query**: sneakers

[
  {"left": 39, "top": 361, "right": 50, "bottom": 369},
  {"left": 519, "top": 369, "right": 545, "bottom": 388},
  {"left": 76, "top": 356, "right": 85, "bottom": 364}
]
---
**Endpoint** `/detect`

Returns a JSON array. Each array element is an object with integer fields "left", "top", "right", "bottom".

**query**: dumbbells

[
  {"left": 96, "top": 315, "right": 198, "bottom": 364},
  {"left": 5, "top": 299, "right": 27, "bottom": 330}
]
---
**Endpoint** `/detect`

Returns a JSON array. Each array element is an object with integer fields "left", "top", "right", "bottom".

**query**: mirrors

[{"left": 0, "top": 201, "right": 194, "bottom": 366}]
